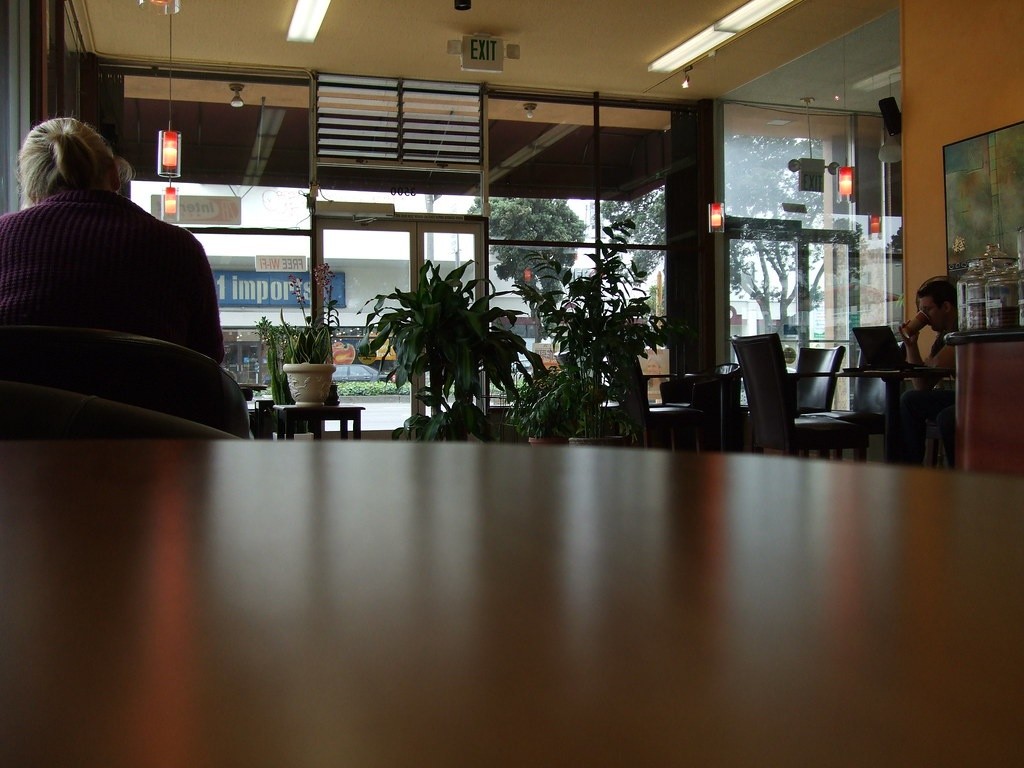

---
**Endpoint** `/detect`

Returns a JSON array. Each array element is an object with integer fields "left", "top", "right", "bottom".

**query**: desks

[
  {"left": 641, "top": 374, "right": 685, "bottom": 450},
  {"left": 272, "top": 404, "right": 366, "bottom": 441},
  {"left": 834, "top": 366, "right": 956, "bottom": 466},
  {"left": 0, "top": 439, "right": 1024, "bottom": 768}
]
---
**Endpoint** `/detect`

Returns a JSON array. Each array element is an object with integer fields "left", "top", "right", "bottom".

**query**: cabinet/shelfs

[{"left": 943, "top": 326, "right": 1024, "bottom": 479}]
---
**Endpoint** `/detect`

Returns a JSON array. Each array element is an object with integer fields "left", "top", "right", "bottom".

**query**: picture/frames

[{"left": 943, "top": 121, "right": 1024, "bottom": 280}]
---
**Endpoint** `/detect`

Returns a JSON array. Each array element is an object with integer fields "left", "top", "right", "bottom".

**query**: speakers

[{"left": 879, "top": 97, "right": 901, "bottom": 136}]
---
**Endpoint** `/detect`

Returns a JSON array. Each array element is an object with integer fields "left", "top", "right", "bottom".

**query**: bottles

[{"left": 955, "top": 226, "right": 1024, "bottom": 331}]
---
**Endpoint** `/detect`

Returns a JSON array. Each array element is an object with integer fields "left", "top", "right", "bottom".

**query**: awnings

[{"left": 729, "top": 269, "right": 824, "bottom": 320}]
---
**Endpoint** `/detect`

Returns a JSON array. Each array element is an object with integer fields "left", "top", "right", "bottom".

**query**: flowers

[{"left": 287, "top": 262, "right": 340, "bottom": 385}]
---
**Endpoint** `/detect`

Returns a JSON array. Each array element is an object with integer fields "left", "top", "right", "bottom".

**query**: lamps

[
  {"left": 138, "top": 0, "right": 182, "bottom": 16},
  {"left": 228, "top": 84, "right": 244, "bottom": 108},
  {"left": 156, "top": 13, "right": 184, "bottom": 178},
  {"left": 286, "top": 0, "right": 331, "bottom": 44},
  {"left": 648, "top": 23, "right": 736, "bottom": 73},
  {"left": 788, "top": 97, "right": 840, "bottom": 193},
  {"left": 867, "top": 215, "right": 882, "bottom": 240},
  {"left": 522, "top": 103, "right": 537, "bottom": 119},
  {"left": 708, "top": 202, "right": 726, "bottom": 234},
  {"left": 164, "top": 179, "right": 180, "bottom": 223},
  {"left": 879, "top": 96, "right": 903, "bottom": 137},
  {"left": 878, "top": 72, "right": 902, "bottom": 163},
  {"left": 714, "top": 0, "right": 794, "bottom": 34},
  {"left": 837, "top": 33, "right": 855, "bottom": 205}
]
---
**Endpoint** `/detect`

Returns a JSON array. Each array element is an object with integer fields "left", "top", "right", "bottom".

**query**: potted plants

[
  {"left": 265, "top": 333, "right": 314, "bottom": 440},
  {"left": 502, "top": 218, "right": 694, "bottom": 448},
  {"left": 280, "top": 307, "right": 337, "bottom": 407}
]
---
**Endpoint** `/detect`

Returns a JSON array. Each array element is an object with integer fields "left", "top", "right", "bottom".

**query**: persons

[
  {"left": 899, "top": 280, "right": 958, "bottom": 469},
  {"left": 645, "top": 357, "right": 668, "bottom": 398},
  {"left": 0, "top": 118, "right": 224, "bottom": 365}
]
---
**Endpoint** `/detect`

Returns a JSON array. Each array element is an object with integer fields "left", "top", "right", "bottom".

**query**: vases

[{"left": 324, "top": 385, "right": 341, "bottom": 407}]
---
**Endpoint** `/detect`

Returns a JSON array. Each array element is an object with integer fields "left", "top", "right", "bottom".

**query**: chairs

[
  {"left": 0, "top": 324, "right": 251, "bottom": 441},
  {"left": 802, "top": 340, "right": 905, "bottom": 461},
  {"left": 925, "top": 422, "right": 942, "bottom": 469},
  {"left": 728, "top": 332, "right": 870, "bottom": 465},
  {"left": 660, "top": 364, "right": 748, "bottom": 454},
  {"left": 797, "top": 347, "right": 846, "bottom": 461}
]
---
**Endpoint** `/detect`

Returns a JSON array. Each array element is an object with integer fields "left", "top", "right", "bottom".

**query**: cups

[{"left": 901, "top": 310, "right": 930, "bottom": 339}]
